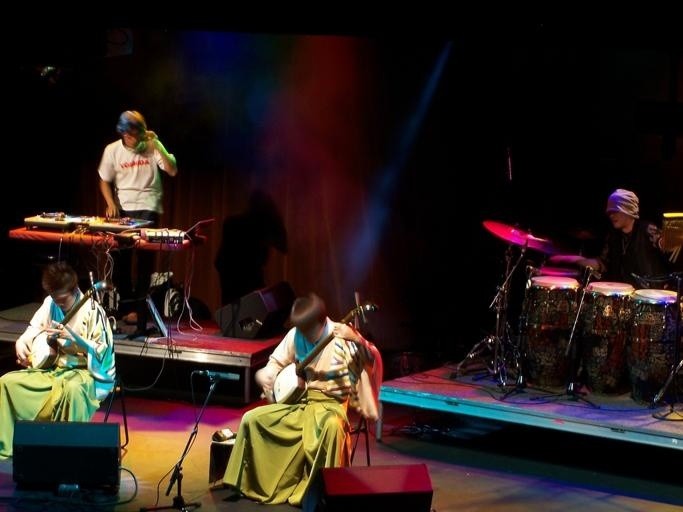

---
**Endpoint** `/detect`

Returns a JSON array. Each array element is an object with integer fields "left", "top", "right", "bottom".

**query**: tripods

[{"left": 450, "top": 255, "right": 601, "bottom": 409}]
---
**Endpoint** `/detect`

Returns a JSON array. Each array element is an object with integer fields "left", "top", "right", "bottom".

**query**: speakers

[
  {"left": 301, "top": 463, "right": 434, "bottom": 512},
  {"left": 12, "top": 420, "right": 122, "bottom": 492},
  {"left": 214, "top": 281, "right": 294, "bottom": 338}
]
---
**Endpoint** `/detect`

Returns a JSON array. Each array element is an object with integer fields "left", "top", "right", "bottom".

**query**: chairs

[{"left": 337, "top": 320, "right": 383, "bottom": 467}]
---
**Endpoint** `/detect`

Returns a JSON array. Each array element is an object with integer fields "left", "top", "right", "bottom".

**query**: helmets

[{"left": 116, "top": 110, "right": 146, "bottom": 134}]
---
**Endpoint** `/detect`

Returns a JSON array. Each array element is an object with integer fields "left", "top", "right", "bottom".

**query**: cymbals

[{"left": 484, "top": 221, "right": 554, "bottom": 252}]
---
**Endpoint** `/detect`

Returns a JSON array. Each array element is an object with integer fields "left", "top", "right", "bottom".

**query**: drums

[
  {"left": 633, "top": 289, "right": 680, "bottom": 405},
  {"left": 537, "top": 266, "right": 580, "bottom": 279},
  {"left": 578, "top": 282, "right": 634, "bottom": 397},
  {"left": 550, "top": 255, "right": 585, "bottom": 263},
  {"left": 521, "top": 275, "right": 580, "bottom": 388}
]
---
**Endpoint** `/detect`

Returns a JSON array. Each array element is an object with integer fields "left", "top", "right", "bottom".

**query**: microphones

[{"left": 194, "top": 370, "right": 240, "bottom": 381}]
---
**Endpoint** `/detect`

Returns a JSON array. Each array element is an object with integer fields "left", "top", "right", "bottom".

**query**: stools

[{"left": 96, "top": 380, "right": 128, "bottom": 449}]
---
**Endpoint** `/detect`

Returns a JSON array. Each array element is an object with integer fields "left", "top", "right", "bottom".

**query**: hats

[{"left": 606, "top": 188, "right": 639, "bottom": 219}]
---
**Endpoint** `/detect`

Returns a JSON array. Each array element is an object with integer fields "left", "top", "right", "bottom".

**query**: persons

[
  {"left": 597, "top": 189, "right": 663, "bottom": 289},
  {"left": 1, "top": 263, "right": 116, "bottom": 465},
  {"left": 221, "top": 291, "right": 383, "bottom": 507},
  {"left": 98, "top": 110, "right": 180, "bottom": 325}
]
